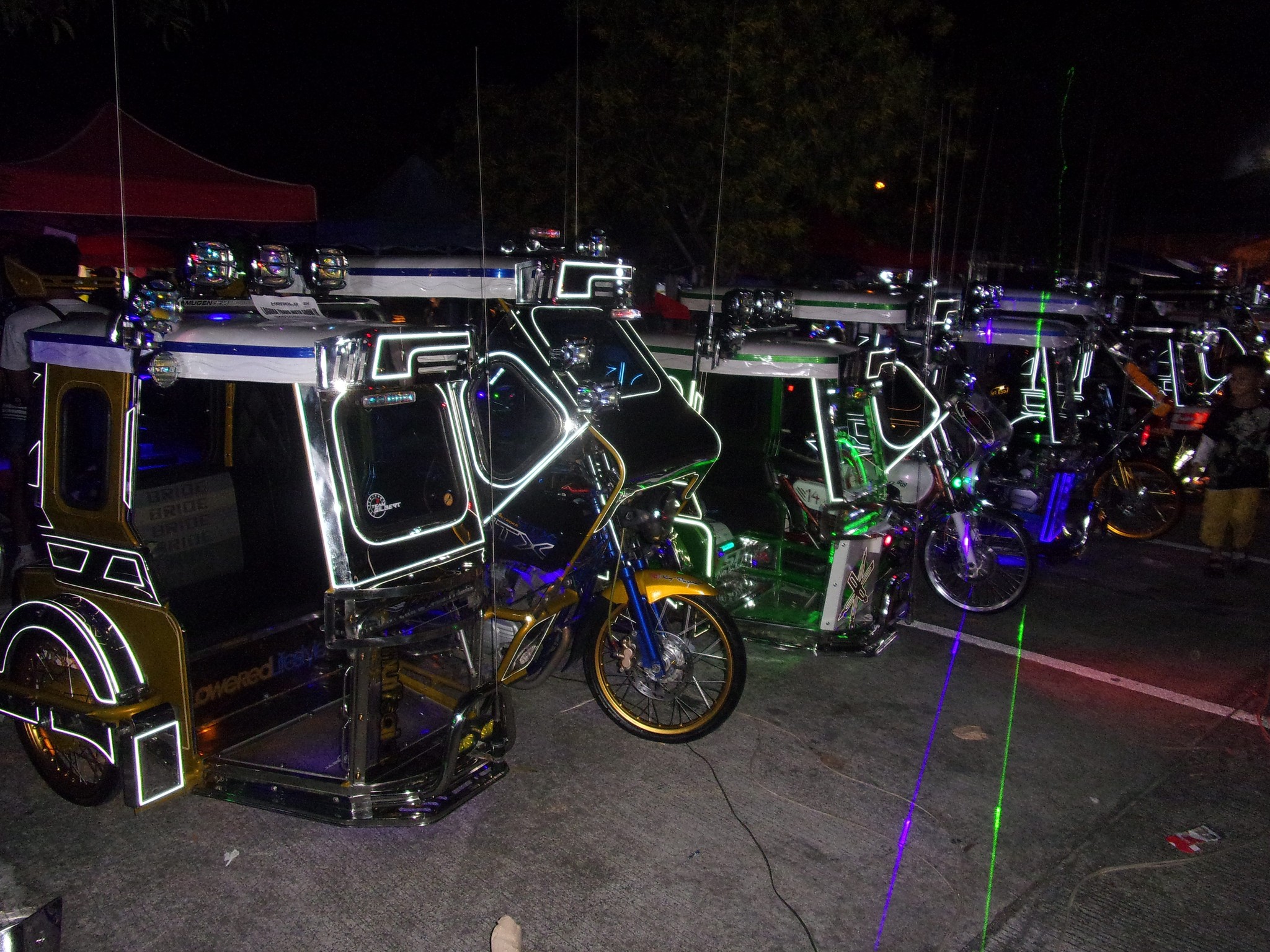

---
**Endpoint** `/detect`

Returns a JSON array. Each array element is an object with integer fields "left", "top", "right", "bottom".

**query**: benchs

[
  {"left": 130, "top": 463, "right": 328, "bottom": 703},
  {"left": 673, "top": 449, "right": 790, "bottom": 582}
]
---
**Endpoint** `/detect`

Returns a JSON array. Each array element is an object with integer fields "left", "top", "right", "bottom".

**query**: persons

[
  {"left": 0, "top": 234, "right": 119, "bottom": 586},
  {"left": 1190, "top": 354, "right": 1270, "bottom": 567}
]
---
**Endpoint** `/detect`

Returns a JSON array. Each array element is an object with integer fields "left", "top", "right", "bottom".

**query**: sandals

[
  {"left": 1208, "top": 558, "right": 1225, "bottom": 578},
  {"left": 1232, "top": 551, "right": 1251, "bottom": 572}
]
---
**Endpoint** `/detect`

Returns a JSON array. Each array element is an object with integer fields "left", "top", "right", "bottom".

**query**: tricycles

[
  {"left": 627, "top": 67, "right": 1269, "bottom": 660},
  {"left": 0, "top": 13, "right": 752, "bottom": 831}
]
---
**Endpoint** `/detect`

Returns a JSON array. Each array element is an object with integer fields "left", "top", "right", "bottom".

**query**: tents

[
  {"left": 782, "top": 208, "right": 962, "bottom": 279},
  {"left": 0, "top": 105, "right": 321, "bottom": 283},
  {"left": 1094, "top": 226, "right": 1270, "bottom": 291}
]
